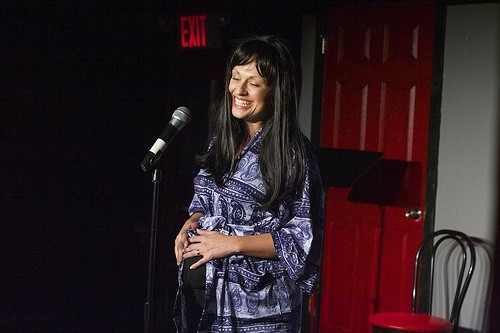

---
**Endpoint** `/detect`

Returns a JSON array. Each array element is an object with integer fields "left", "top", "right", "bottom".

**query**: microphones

[{"left": 140, "top": 107, "right": 192, "bottom": 172}]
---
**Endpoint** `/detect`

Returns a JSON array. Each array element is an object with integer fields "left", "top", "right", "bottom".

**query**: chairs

[{"left": 368, "top": 229, "right": 478, "bottom": 333}]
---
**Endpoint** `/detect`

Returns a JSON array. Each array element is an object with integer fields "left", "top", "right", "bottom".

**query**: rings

[{"left": 197, "top": 250, "right": 200, "bottom": 256}]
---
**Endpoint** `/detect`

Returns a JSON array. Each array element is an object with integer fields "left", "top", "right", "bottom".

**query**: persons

[{"left": 169, "top": 36, "right": 326, "bottom": 333}]
